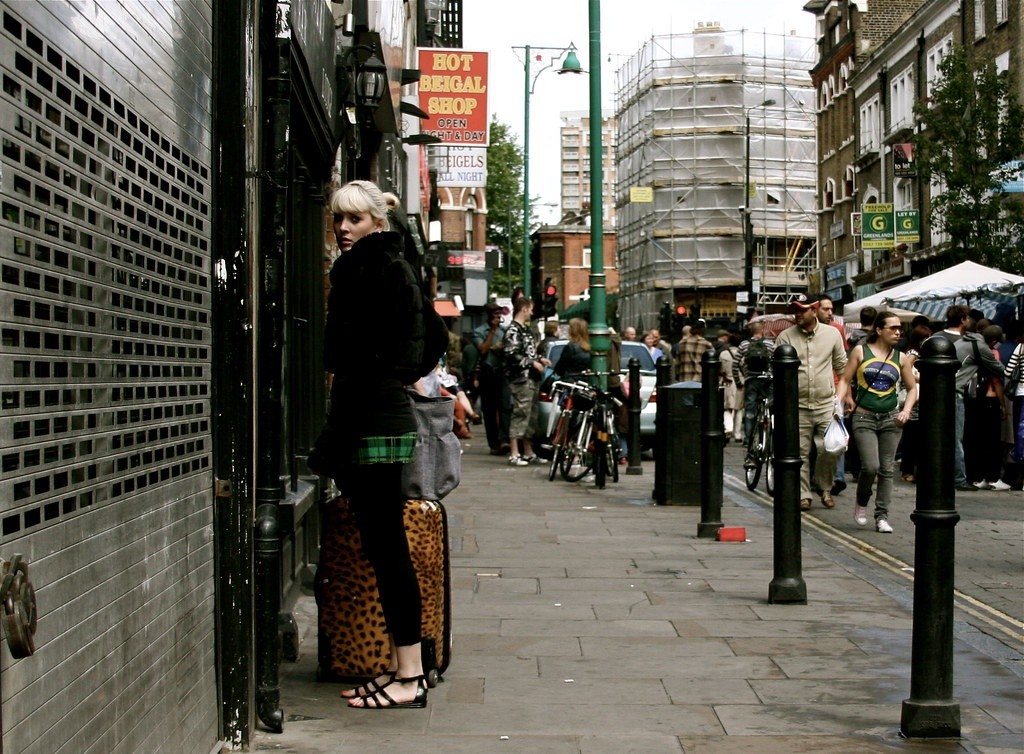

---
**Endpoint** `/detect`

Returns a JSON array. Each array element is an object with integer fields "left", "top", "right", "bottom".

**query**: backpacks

[{"left": 745, "top": 340, "right": 770, "bottom": 372}]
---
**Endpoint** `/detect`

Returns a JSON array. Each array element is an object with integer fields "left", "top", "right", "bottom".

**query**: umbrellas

[
  {"left": 844, "top": 259, "right": 1024, "bottom": 310},
  {"left": 748, "top": 313, "right": 862, "bottom": 341}
]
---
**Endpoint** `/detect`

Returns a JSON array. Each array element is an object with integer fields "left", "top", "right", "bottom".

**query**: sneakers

[
  {"left": 508, "top": 452, "right": 529, "bottom": 467},
  {"left": 875, "top": 517, "right": 893, "bottom": 533},
  {"left": 988, "top": 479, "right": 1011, "bottom": 491},
  {"left": 855, "top": 501, "right": 867, "bottom": 526},
  {"left": 744, "top": 454, "right": 757, "bottom": 470},
  {"left": 821, "top": 490, "right": 835, "bottom": 508},
  {"left": 972, "top": 478, "right": 987, "bottom": 489},
  {"left": 800, "top": 498, "right": 811, "bottom": 510},
  {"left": 522, "top": 454, "right": 548, "bottom": 465}
]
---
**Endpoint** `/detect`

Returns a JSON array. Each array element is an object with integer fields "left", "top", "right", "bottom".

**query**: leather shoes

[{"left": 955, "top": 482, "right": 978, "bottom": 491}]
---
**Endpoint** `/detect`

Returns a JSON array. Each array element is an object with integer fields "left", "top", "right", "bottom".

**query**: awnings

[{"left": 560, "top": 294, "right": 621, "bottom": 319}]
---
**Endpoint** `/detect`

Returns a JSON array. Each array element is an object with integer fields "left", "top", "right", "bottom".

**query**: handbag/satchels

[
  {"left": 402, "top": 388, "right": 461, "bottom": 501},
  {"left": 964, "top": 365, "right": 991, "bottom": 402},
  {"left": 822, "top": 405, "right": 850, "bottom": 456},
  {"left": 844, "top": 410, "right": 855, "bottom": 435},
  {"left": 542, "top": 370, "right": 562, "bottom": 397},
  {"left": 1003, "top": 364, "right": 1021, "bottom": 402}
]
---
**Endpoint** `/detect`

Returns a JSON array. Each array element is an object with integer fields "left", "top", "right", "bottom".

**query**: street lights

[
  {"left": 743, "top": 99, "right": 777, "bottom": 293},
  {"left": 524, "top": 44, "right": 582, "bottom": 300}
]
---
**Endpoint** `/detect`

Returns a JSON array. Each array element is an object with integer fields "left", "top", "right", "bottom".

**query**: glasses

[{"left": 883, "top": 326, "right": 902, "bottom": 331}]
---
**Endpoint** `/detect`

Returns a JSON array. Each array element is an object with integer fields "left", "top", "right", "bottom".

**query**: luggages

[{"left": 316, "top": 496, "right": 450, "bottom": 688}]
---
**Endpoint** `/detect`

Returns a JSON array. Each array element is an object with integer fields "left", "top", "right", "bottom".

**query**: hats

[
  {"left": 984, "top": 324, "right": 1004, "bottom": 343},
  {"left": 716, "top": 330, "right": 732, "bottom": 338},
  {"left": 792, "top": 292, "right": 819, "bottom": 309}
]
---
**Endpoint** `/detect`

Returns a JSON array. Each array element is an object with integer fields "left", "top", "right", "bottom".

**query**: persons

[
  {"left": 471, "top": 296, "right": 592, "bottom": 466},
  {"left": 931, "top": 303, "right": 1024, "bottom": 492},
  {"left": 304, "top": 179, "right": 429, "bottom": 711},
  {"left": 607, "top": 318, "right": 746, "bottom": 466},
  {"left": 404, "top": 331, "right": 482, "bottom": 439},
  {"left": 732, "top": 293, "right": 933, "bottom": 533}
]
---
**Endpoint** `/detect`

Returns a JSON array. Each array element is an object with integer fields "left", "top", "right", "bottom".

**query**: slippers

[{"left": 902, "top": 474, "right": 916, "bottom": 481}]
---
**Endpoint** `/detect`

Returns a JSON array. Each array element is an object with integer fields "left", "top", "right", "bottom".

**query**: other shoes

[
  {"left": 619, "top": 458, "right": 626, "bottom": 463},
  {"left": 735, "top": 439, "right": 743, "bottom": 443},
  {"left": 491, "top": 442, "right": 511, "bottom": 456},
  {"left": 726, "top": 433, "right": 731, "bottom": 438},
  {"left": 830, "top": 480, "right": 846, "bottom": 496},
  {"left": 468, "top": 413, "right": 479, "bottom": 418},
  {"left": 457, "top": 431, "right": 471, "bottom": 439}
]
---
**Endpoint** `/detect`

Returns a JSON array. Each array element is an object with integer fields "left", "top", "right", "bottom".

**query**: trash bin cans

[{"left": 654, "top": 381, "right": 724, "bottom": 506}]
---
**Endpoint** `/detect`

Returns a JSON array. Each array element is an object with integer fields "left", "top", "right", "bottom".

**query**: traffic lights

[{"left": 544, "top": 285, "right": 557, "bottom": 318}]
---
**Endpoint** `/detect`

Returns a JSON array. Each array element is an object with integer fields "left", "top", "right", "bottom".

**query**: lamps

[
  {"left": 336, "top": 13, "right": 355, "bottom": 38},
  {"left": 337, "top": 40, "right": 387, "bottom": 128}
]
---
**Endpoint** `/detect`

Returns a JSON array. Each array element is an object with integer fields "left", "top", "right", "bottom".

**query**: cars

[{"left": 528, "top": 337, "right": 658, "bottom": 461}]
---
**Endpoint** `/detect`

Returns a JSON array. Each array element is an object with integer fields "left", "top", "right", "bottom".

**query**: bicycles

[
  {"left": 743, "top": 370, "right": 775, "bottom": 496},
  {"left": 546, "top": 369, "right": 628, "bottom": 484}
]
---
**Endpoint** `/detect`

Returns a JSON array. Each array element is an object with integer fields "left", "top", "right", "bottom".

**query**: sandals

[{"left": 341, "top": 668, "right": 428, "bottom": 709}]
